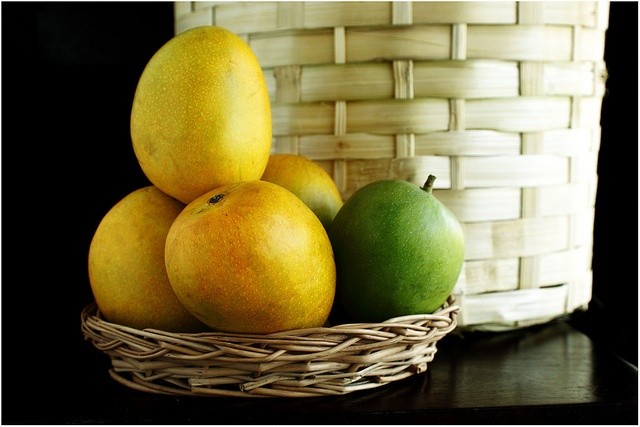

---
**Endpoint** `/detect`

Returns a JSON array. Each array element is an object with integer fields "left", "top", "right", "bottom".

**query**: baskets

[
  {"left": 173, "top": 2, "right": 613, "bottom": 331},
  {"left": 73, "top": 287, "right": 464, "bottom": 401}
]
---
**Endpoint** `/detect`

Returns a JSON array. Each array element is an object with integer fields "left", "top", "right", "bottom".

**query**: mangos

[
  {"left": 164, "top": 181, "right": 337, "bottom": 334},
  {"left": 261, "top": 154, "right": 346, "bottom": 230},
  {"left": 131, "top": 28, "right": 271, "bottom": 203},
  {"left": 89, "top": 188, "right": 191, "bottom": 333},
  {"left": 324, "top": 174, "right": 465, "bottom": 320}
]
